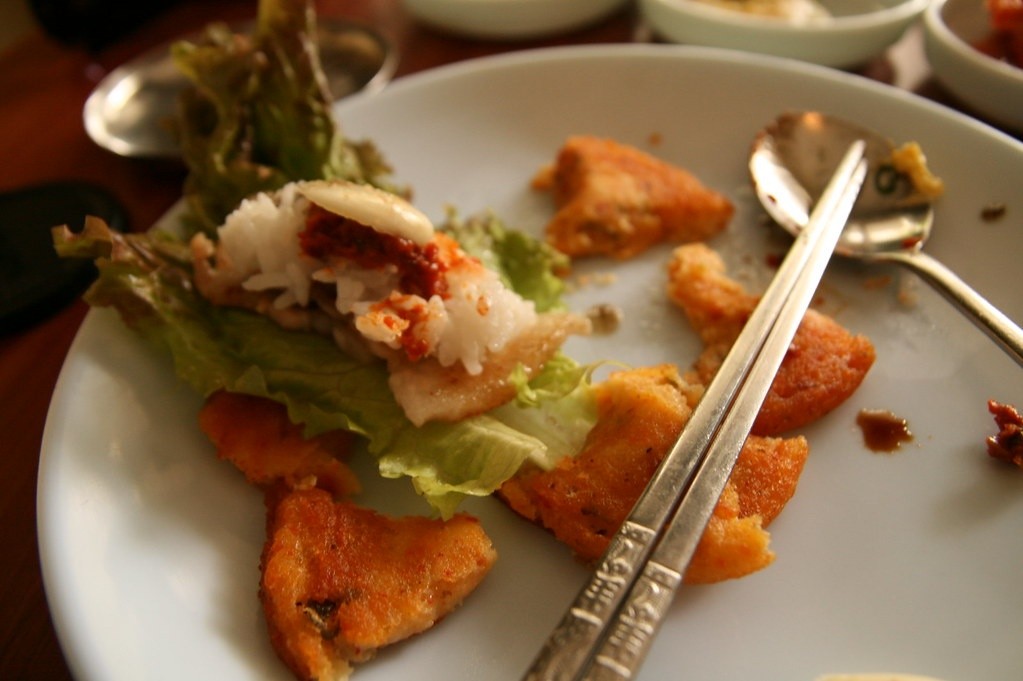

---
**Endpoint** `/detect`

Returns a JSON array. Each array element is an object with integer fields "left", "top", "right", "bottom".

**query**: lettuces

[{"left": 49, "top": 0, "right": 599, "bottom": 522}]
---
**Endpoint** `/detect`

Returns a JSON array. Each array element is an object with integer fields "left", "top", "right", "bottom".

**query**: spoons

[{"left": 749, "top": 110, "right": 1023, "bottom": 370}]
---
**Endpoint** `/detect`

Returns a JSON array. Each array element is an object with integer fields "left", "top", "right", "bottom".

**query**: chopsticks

[{"left": 521, "top": 138, "right": 869, "bottom": 681}]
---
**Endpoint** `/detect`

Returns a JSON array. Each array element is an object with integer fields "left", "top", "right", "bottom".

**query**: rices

[{"left": 190, "top": 180, "right": 538, "bottom": 376}]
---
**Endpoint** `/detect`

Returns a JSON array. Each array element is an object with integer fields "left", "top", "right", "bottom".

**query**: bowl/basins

[
  {"left": 643, "top": 0, "right": 932, "bottom": 72},
  {"left": 925, "top": 0, "right": 1023, "bottom": 127},
  {"left": 408, "top": 0, "right": 619, "bottom": 38}
]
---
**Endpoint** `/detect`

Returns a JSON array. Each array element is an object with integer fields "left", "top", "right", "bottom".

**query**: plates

[
  {"left": 79, "top": 18, "right": 396, "bottom": 159},
  {"left": 35, "top": 44, "right": 1023, "bottom": 681}
]
